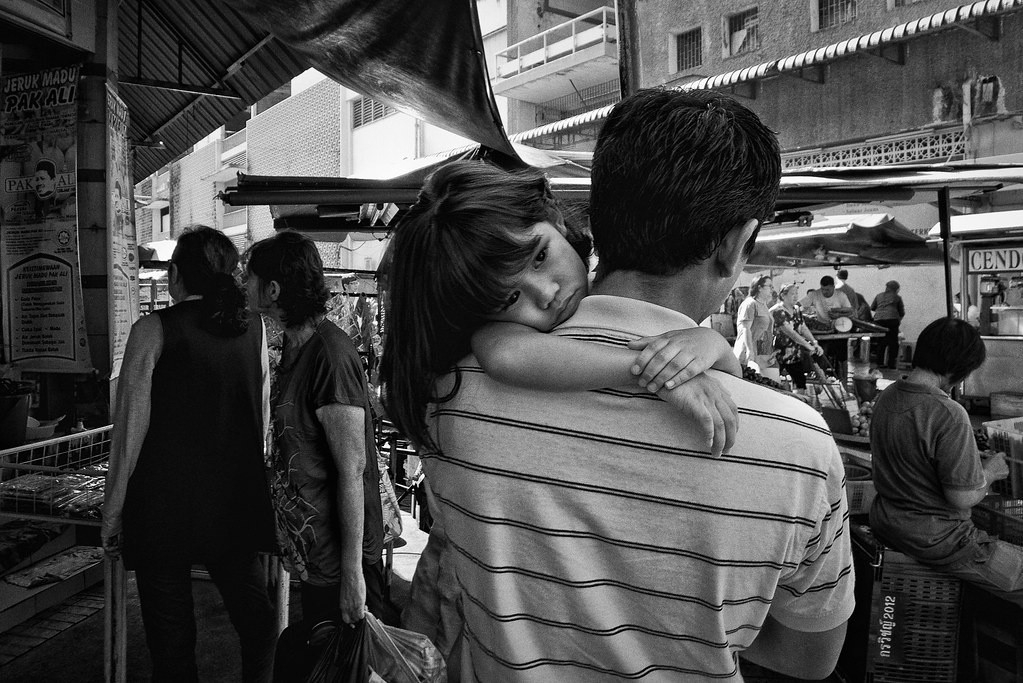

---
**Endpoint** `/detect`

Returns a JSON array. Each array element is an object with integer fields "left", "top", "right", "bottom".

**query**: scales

[{"left": 828, "top": 306, "right": 854, "bottom": 333}]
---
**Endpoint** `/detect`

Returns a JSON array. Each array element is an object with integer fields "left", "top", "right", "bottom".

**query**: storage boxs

[
  {"left": 982, "top": 416, "right": 1023, "bottom": 442},
  {"left": 845, "top": 480, "right": 878, "bottom": 514},
  {"left": 698, "top": 314, "right": 734, "bottom": 337},
  {"left": 849, "top": 523, "right": 965, "bottom": 683}
]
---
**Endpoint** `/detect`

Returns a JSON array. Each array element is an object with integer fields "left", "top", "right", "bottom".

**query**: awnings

[
  {"left": 391, "top": 1, "right": 1023, "bottom": 180},
  {"left": 115, "top": 1, "right": 314, "bottom": 185}
]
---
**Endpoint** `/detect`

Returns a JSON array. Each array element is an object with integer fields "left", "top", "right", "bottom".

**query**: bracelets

[{"left": 811, "top": 340, "right": 818, "bottom": 344}]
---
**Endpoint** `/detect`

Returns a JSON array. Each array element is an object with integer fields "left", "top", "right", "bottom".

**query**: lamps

[{"left": 131, "top": 133, "right": 166, "bottom": 149}]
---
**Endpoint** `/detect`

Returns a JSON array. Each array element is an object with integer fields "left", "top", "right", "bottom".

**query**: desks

[{"left": 725, "top": 334, "right": 886, "bottom": 391}]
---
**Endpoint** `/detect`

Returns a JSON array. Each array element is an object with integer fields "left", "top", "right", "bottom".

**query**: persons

[
  {"left": 871, "top": 281, "right": 905, "bottom": 369},
  {"left": 870, "top": 317, "right": 1023, "bottom": 604},
  {"left": 35, "top": 162, "right": 122, "bottom": 226},
  {"left": 382, "top": 161, "right": 744, "bottom": 456},
  {"left": 770, "top": 284, "right": 824, "bottom": 390},
  {"left": 955, "top": 291, "right": 1010, "bottom": 336},
  {"left": 245, "top": 233, "right": 384, "bottom": 683},
  {"left": 102, "top": 227, "right": 273, "bottom": 683},
  {"left": 734, "top": 276, "right": 776, "bottom": 373},
  {"left": 399, "top": 88, "right": 856, "bottom": 683},
  {"left": 726, "top": 275, "right": 874, "bottom": 377}
]
